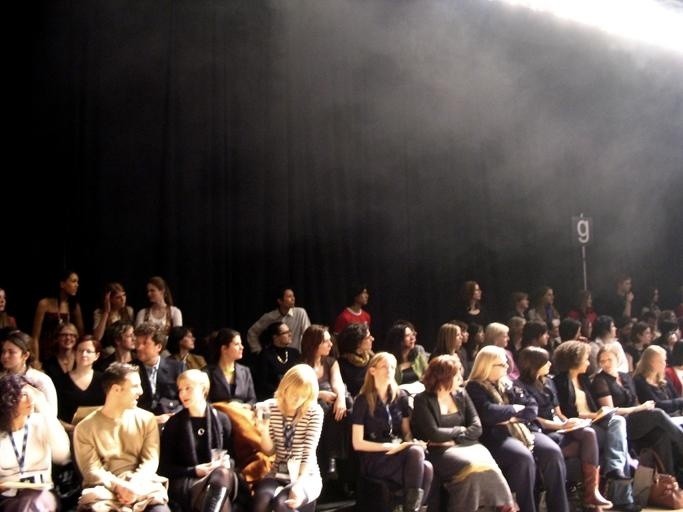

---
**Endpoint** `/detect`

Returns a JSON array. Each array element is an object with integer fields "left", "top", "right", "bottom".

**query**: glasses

[{"left": 282, "top": 328, "right": 291, "bottom": 335}]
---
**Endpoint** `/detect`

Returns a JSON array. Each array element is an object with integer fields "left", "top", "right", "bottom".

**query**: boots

[{"left": 582, "top": 464, "right": 613, "bottom": 508}]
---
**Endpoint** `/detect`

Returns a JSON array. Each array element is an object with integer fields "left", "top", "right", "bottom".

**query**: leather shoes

[
  {"left": 614, "top": 503, "right": 641, "bottom": 512},
  {"left": 605, "top": 470, "right": 633, "bottom": 484}
]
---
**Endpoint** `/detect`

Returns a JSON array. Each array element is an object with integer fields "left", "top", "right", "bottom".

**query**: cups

[{"left": 210, "top": 447, "right": 228, "bottom": 471}]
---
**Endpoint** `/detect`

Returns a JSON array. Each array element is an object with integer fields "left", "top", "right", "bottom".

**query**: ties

[{"left": 147, "top": 367, "right": 156, "bottom": 394}]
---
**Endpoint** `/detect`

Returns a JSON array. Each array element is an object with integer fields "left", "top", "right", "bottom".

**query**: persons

[{"left": 1, "top": 266, "right": 683, "bottom": 512}]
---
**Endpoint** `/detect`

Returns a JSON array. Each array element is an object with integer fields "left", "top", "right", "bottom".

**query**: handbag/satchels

[
  {"left": 648, "top": 474, "right": 682, "bottom": 510},
  {"left": 505, "top": 417, "right": 534, "bottom": 453},
  {"left": 54, "top": 460, "right": 82, "bottom": 508},
  {"left": 632, "top": 455, "right": 657, "bottom": 508}
]
